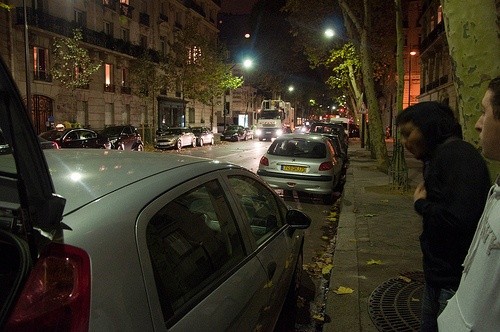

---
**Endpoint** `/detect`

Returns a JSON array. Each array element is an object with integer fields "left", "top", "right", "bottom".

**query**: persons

[
  {"left": 397, "top": 101, "right": 494, "bottom": 332},
  {"left": 434, "top": 77, "right": 500, "bottom": 332}
]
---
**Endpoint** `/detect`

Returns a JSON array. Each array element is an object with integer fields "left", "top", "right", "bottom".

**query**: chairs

[{"left": 312, "top": 144, "right": 326, "bottom": 154}]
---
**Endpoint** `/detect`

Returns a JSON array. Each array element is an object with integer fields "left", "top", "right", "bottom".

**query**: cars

[
  {"left": 257, "top": 122, "right": 348, "bottom": 194},
  {"left": 98, "top": 126, "right": 144, "bottom": 151},
  {"left": 154, "top": 127, "right": 196, "bottom": 150},
  {"left": 40, "top": 127, "right": 97, "bottom": 148},
  {"left": 0, "top": 136, "right": 59, "bottom": 153},
  {"left": 220, "top": 125, "right": 253, "bottom": 141},
  {"left": 191, "top": 126, "right": 214, "bottom": 147}
]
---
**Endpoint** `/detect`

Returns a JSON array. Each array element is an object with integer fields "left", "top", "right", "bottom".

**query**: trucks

[{"left": 257, "top": 100, "right": 294, "bottom": 140}]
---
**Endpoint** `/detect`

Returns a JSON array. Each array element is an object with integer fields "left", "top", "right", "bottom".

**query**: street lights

[
  {"left": 408, "top": 50, "right": 417, "bottom": 107},
  {"left": 324, "top": 28, "right": 365, "bottom": 148}
]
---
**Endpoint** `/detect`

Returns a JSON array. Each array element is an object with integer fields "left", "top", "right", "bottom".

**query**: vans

[{"left": 0, "top": 149, "right": 312, "bottom": 332}]
